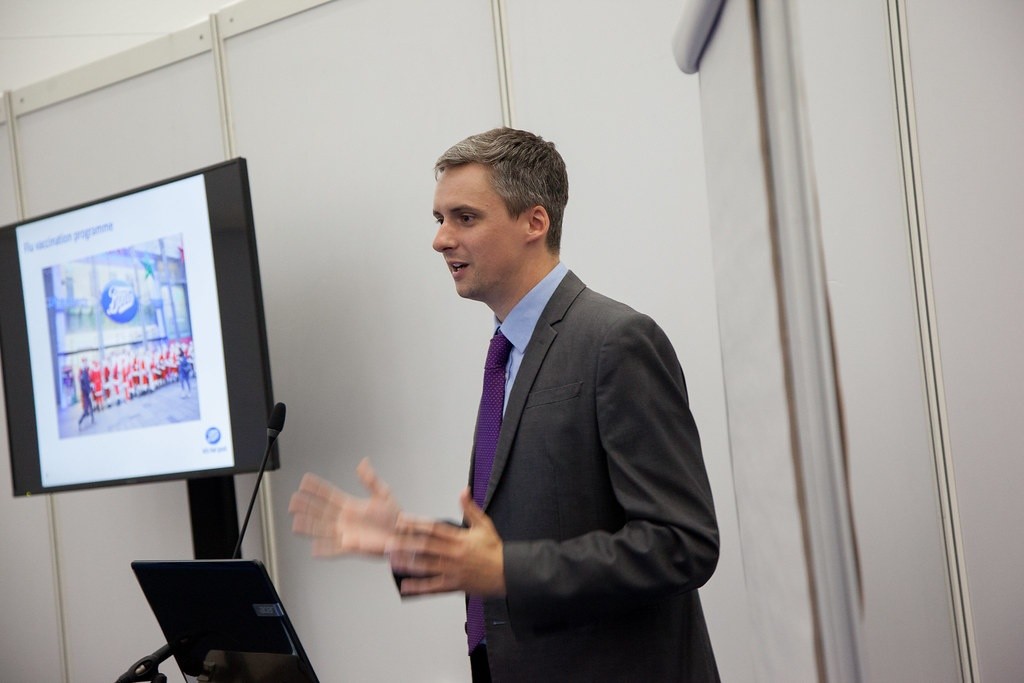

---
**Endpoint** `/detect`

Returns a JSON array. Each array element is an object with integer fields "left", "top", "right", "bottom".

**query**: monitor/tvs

[{"left": 0, "top": 157, "right": 279, "bottom": 502}]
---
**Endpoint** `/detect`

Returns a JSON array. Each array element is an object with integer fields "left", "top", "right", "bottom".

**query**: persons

[
  {"left": 77, "top": 367, "right": 94, "bottom": 424},
  {"left": 176, "top": 347, "right": 192, "bottom": 399},
  {"left": 289, "top": 127, "right": 722, "bottom": 683}
]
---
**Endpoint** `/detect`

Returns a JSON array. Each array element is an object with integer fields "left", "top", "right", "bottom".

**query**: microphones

[{"left": 231, "top": 401, "right": 286, "bottom": 559}]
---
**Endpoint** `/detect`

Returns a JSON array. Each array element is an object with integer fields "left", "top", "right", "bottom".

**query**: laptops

[{"left": 128, "top": 557, "right": 322, "bottom": 683}]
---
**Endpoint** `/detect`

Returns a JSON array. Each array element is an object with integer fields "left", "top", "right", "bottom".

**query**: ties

[{"left": 464, "top": 333, "right": 520, "bottom": 657}]
